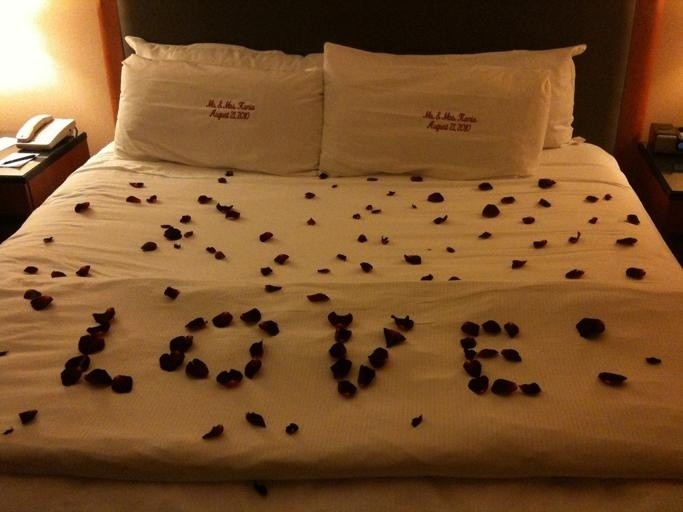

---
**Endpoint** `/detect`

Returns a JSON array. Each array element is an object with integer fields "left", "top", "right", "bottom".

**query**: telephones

[{"left": 16, "top": 114, "right": 75, "bottom": 150}]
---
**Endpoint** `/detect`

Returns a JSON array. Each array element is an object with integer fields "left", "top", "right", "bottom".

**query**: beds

[{"left": 0, "top": 1, "right": 682, "bottom": 511}]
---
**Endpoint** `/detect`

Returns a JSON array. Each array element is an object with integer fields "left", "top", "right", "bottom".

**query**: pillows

[
  {"left": 124, "top": 35, "right": 324, "bottom": 73},
  {"left": 319, "top": 42, "right": 585, "bottom": 192},
  {"left": 114, "top": 53, "right": 323, "bottom": 183}
]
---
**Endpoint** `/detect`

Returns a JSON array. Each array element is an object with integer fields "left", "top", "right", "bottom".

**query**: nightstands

[
  {"left": 639, "top": 140, "right": 682, "bottom": 241},
  {"left": 0, "top": 131, "right": 90, "bottom": 212}
]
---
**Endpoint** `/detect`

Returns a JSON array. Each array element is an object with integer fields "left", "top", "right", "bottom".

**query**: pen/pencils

[{"left": 4, "top": 155, "right": 36, "bottom": 164}]
